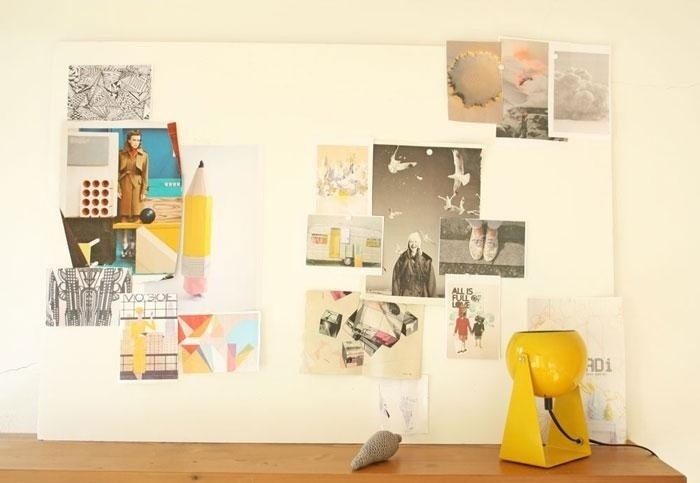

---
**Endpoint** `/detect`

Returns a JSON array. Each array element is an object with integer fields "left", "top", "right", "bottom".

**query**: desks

[{"left": 0, "top": 438, "right": 686, "bottom": 483}]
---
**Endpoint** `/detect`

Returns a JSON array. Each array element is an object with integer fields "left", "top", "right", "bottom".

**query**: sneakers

[{"left": 469, "top": 229, "right": 499, "bottom": 262}]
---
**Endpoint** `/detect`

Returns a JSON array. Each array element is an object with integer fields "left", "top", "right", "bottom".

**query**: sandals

[{"left": 122, "top": 246, "right": 134, "bottom": 258}]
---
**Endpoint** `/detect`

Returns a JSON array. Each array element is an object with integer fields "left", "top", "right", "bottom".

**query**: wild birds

[
  {"left": 385, "top": 207, "right": 401, "bottom": 220},
  {"left": 447, "top": 149, "right": 471, "bottom": 193},
  {"left": 388, "top": 145, "right": 418, "bottom": 174}
]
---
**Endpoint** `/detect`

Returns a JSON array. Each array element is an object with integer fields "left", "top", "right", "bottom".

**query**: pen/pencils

[{"left": 181, "top": 160, "right": 213, "bottom": 296}]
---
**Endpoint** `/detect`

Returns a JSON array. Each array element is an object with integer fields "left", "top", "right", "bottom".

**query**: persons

[
  {"left": 119, "top": 131, "right": 149, "bottom": 257},
  {"left": 392, "top": 233, "right": 437, "bottom": 299},
  {"left": 453, "top": 304, "right": 484, "bottom": 353},
  {"left": 466, "top": 220, "right": 501, "bottom": 262}
]
